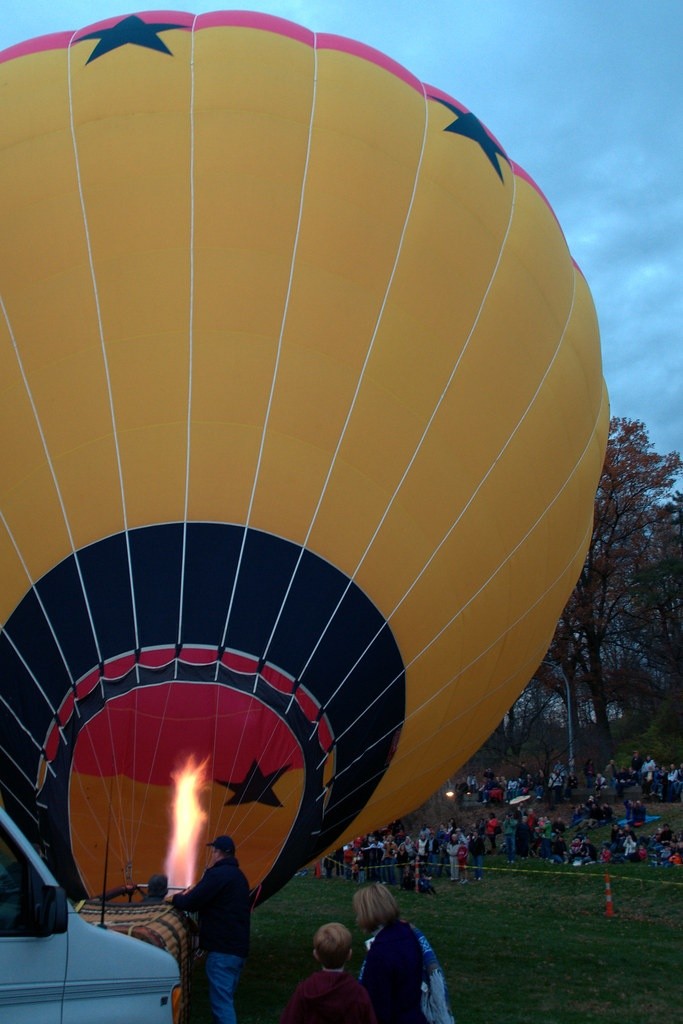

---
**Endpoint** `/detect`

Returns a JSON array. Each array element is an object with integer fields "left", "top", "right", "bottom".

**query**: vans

[{"left": 0, "top": 803, "right": 183, "bottom": 1024}]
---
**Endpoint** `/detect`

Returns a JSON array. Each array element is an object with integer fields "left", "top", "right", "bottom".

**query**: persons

[
  {"left": 649, "top": 823, "right": 683, "bottom": 861},
  {"left": 502, "top": 801, "right": 567, "bottom": 866},
  {"left": 548, "top": 760, "right": 578, "bottom": 803},
  {"left": 454, "top": 761, "right": 547, "bottom": 804},
  {"left": 570, "top": 794, "right": 612, "bottom": 831},
  {"left": 279, "top": 922, "right": 378, "bottom": 1024},
  {"left": 139, "top": 836, "right": 252, "bottom": 1024},
  {"left": 611, "top": 825, "right": 641, "bottom": 861},
  {"left": 354, "top": 885, "right": 453, "bottom": 1024},
  {"left": 486, "top": 813, "right": 498, "bottom": 854},
  {"left": 323, "top": 818, "right": 485, "bottom": 885},
  {"left": 624, "top": 798, "right": 646, "bottom": 824},
  {"left": 569, "top": 833, "right": 597, "bottom": 865},
  {"left": 583, "top": 759, "right": 595, "bottom": 789},
  {"left": 629, "top": 751, "right": 683, "bottom": 804},
  {"left": 594, "top": 760, "right": 638, "bottom": 799}
]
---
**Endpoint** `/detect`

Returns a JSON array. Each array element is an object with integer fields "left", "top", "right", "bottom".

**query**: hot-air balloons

[{"left": 1, "top": 2, "right": 610, "bottom": 1000}]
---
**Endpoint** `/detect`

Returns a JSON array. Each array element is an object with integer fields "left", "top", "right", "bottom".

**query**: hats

[
  {"left": 148, "top": 874, "right": 167, "bottom": 896},
  {"left": 206, "top": 835, "right": 235, "bottom": 854}
]
